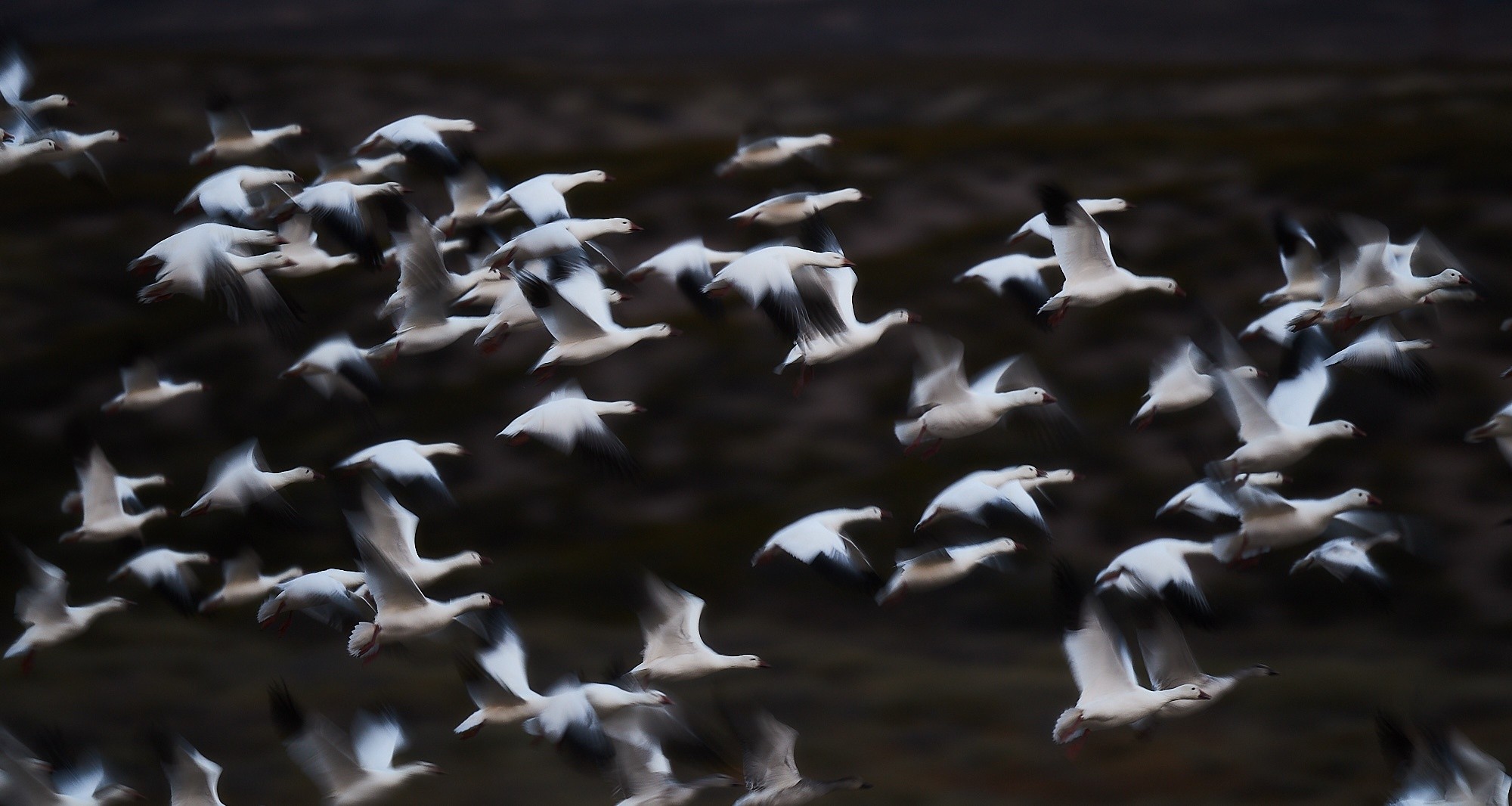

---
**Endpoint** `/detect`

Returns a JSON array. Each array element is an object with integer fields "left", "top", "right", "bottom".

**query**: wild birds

[
  {"left": 622, "top": 132, "right": 1195, "bottom": 448},
  {"left": 747, "top": 228, "right": 1476, "bottom": 746},
  {"left": 0, "top": 52, "right": 133, "bottom": 173},
  {"left": 7, "top": 439, "right": 899, "bottom": 806},
  {"left": 98, "top": 113, "right": 684, "bottom": 459}
]
